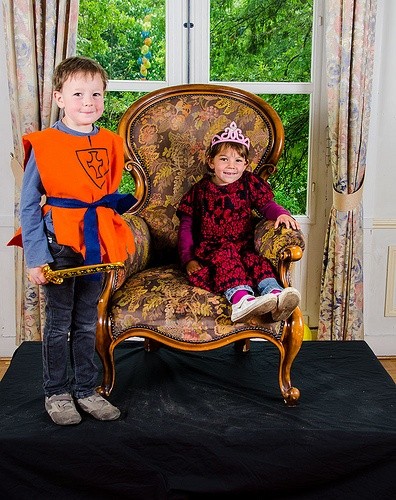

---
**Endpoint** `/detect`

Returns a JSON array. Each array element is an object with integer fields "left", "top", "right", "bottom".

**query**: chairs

[{"left": 96, "top": 83, "right": 307, "bottom": 407}]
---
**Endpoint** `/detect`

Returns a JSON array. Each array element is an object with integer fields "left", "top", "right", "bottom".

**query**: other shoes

[
  {"left": 230, "top": 292, "right": 278, "bottom": 323},
  {"left": 271, "top": 287, "right": 301, "bottom": 321}
]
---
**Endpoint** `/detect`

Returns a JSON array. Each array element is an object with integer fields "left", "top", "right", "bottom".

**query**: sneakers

[
  {"left": 45, "top": 393, "right": 81, "bottom": 425},
  {"left": 76, "top": 393, "right": 121, "bottom": 420}
]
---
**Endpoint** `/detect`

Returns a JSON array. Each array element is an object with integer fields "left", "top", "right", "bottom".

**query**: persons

[
  {"left": 177, "top": 121, "right": 301, "bottom": 321},
  {"left": 6, "top": 57, "right": 138, "bottom": 424}
]
